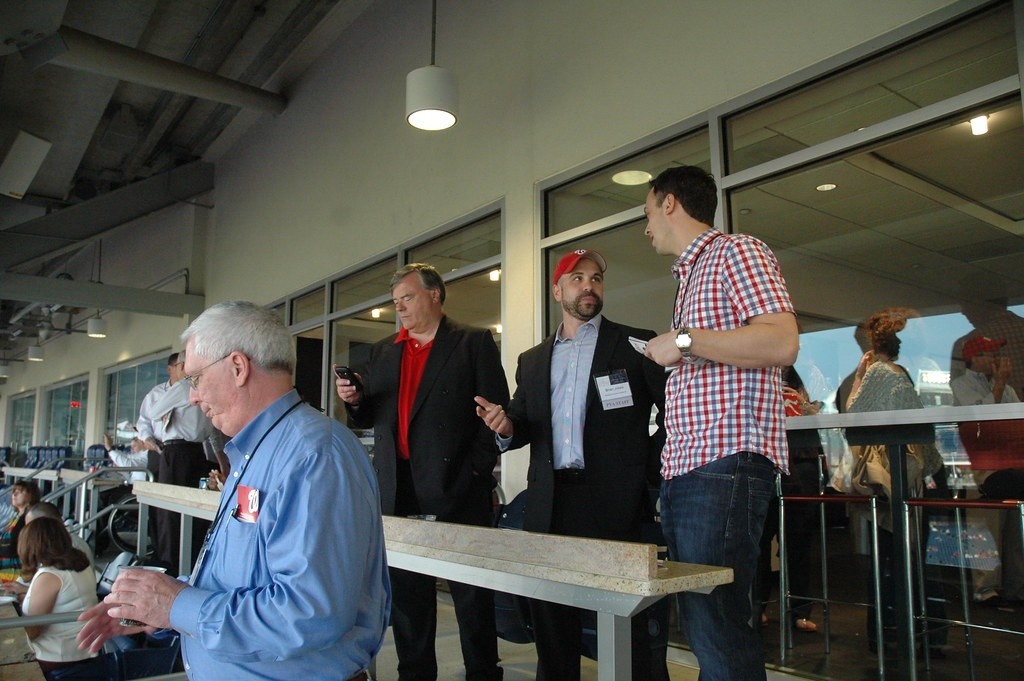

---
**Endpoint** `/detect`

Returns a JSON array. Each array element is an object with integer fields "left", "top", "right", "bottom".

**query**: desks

[
  {"left": 5, "top": 463, "right": 57, "bottom": 516},
  {"left": 764, "top": 402, "right": 1024, "bottom": 660},
  {"left": 58, "top": 468, "right": 124, "bottom": 544},
  {"left": 128, "top": 478, "right": 733, "bottom": 681}
]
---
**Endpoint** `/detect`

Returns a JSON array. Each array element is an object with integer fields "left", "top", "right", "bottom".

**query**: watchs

[{"left": 675, "top": 328, "right": 693, "bottom": 357}]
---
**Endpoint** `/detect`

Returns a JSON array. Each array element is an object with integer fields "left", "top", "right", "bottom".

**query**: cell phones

[{"left": 335, "top": 367, "right": 363, "bottom": 392}]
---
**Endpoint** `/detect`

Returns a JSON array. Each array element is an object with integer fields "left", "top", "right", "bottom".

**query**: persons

[
  {"left": 0, "top": 348, "right": 232, "bottom": 681},
  {"left": 645, "top": 165, "right": 799, "bottom": 681},
  {"left": 335, "top": 262, "right": 511, "bottom": 681},
  {"left": 756, "top": 307, "right": 1023, "bottom": 654},
  {"left": 943, "top": 263, "right": 1023, "bottom": 604},
  {"left": 75, "top": 300, "right": 390, "bottom": 681},
  {"left": 475, "top": 248, "right": 668, "bottom": 681}
]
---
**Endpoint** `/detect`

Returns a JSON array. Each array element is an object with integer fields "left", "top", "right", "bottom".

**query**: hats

[
  {"left": 962, "top": 334, "right": 1008, "bottom": 362},
  {"left": 553, "top": 248, "right": 607, "bottom": 285}
]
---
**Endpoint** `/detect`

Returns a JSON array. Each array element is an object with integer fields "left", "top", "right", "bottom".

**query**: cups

[{"left": 117, "top": 565, "right": 167, "bottom": 628}]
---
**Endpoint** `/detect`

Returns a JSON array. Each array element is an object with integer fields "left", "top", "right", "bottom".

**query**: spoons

[{"left": 211, "top": 469, "right": 223, "bottom": 491}]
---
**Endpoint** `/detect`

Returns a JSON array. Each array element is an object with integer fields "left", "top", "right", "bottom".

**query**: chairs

[
  {"left": 0, "top": 444, "right": 132, "bottom": 483},
  {"left": 88, "top": 552, "right": 138, "bottom": 599}
]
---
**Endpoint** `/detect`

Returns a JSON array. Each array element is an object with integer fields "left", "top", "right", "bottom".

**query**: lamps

[
  {"left": 87, "top": 238, "right": 107, "bottom": 338},
  {"left": 0, "top": 349, "right": 11, "bottom": 378},
  {"left": 28, "top": 314, "right": 43, "bottom": 361},
  {"left": 406, "top": 0, "right": 458, "bottom": 131},
  {"left": 965, "top": 111, "right": 992, "bottom": 137}
]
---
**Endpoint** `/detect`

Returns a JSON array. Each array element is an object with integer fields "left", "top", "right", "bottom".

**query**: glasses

[
  {"left": 184, "top": 354, "right": 228, "bottom": 390},
  {"left": 173, "top": 362, "right": 183, "bottom": 371},
  {"left": 978, "top": 350, "right": 1009, "bottom": 361}
]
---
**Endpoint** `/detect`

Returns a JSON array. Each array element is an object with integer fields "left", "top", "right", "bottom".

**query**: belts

[{"left": 163, "top": 439, "right": 197, "bottom": 445}]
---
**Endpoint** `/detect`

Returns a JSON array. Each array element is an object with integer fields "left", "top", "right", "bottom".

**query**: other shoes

[
  {"left": 795, "top": 618, "right": 817, "bottom": 631},
  {"left": 974, "top": 583, "right": 999, "bottom": 602},
  {"left": 159, "top": 561, "right": 171, "bottom": 574},
  {"left": 762, "top": 613, "right": 768, "bottom": 627}
]
